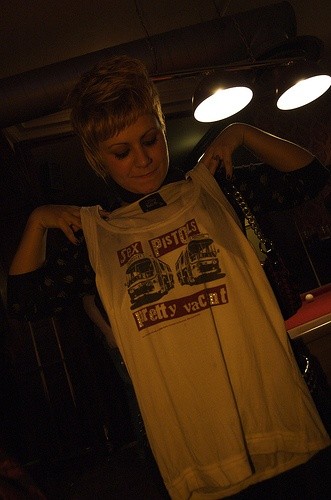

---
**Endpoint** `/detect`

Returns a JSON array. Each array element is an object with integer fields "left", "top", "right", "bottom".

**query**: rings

[{"left": 212, "top": 154, "right": 221, "bottom": 161}]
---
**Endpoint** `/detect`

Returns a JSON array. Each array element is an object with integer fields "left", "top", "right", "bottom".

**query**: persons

[
  {"left": 7, "top": 52, "right": 331, "bottom": 500},
  {"left": 78, "top": 242, "right": 145, "bottom": 456}
]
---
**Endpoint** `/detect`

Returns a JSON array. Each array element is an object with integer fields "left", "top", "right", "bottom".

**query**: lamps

[
  {"left": 191, "top": 71, "right": 254, "bottom": 123},
  {"left": 272, "top": 61, "right": 331, "bottom": 111}
]
